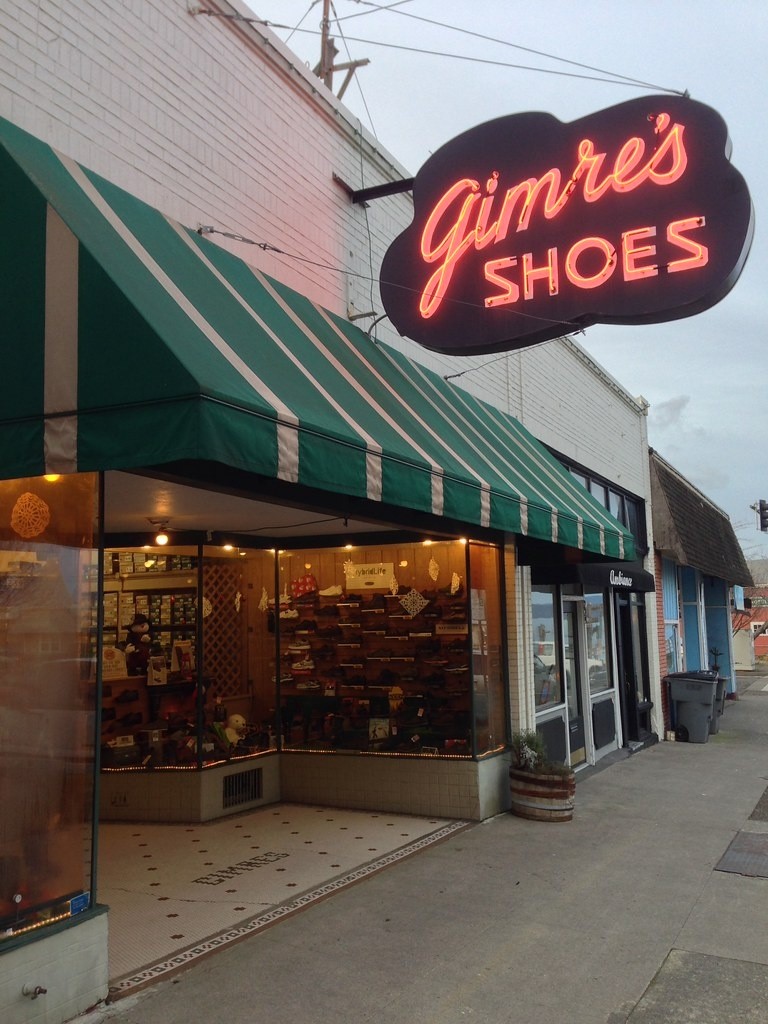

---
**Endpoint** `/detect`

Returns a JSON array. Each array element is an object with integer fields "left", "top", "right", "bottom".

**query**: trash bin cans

[{"left": 663, "top": 670, "right": 729, "bottom": 744}]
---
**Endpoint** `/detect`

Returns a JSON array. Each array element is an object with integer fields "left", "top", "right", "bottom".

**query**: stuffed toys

[
  {"left": 119, "top": 614, "right": 152, "bottom": 676},
  {"left": 225, "top": 714, "right": 246, "bottom": 746}
]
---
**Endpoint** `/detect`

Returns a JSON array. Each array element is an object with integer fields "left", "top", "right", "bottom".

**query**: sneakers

[{"left": 270, "top": 585, "right": 470, "bottom": 727}]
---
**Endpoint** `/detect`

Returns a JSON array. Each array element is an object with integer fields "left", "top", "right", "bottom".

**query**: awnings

[
  {"left": 526, "top": 546, "right": 655, "bottom": 598},
  {"left": 0, "top": 116, "right": 634, "bottom": 568}
]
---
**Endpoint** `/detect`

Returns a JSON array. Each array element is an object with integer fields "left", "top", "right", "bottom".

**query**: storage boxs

[{"left": 90, "top": 551, "right": 197, "bottom": 659}]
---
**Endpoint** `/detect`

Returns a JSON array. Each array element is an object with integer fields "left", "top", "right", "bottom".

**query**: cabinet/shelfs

[{"left": 82, "top": 547, "right": 201, "bottom": 664}]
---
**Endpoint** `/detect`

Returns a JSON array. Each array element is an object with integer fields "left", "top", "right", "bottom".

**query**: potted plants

[
  {"left": 502, "top": 726, "right": 576, "bottom": 824},
  {"left": 710, "top": 646, "right": 724, "bottom": 672}
]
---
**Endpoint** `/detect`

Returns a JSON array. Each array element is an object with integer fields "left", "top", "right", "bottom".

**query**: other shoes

[
  {"left": 101, "top": 706, "right": 114, "bottom": 720},
  {"left": 115, "top": 689, "right": 138, "bottom": 702},
  {"left": 119, "top": 712, "right": 142, "bottom": 727},
  {"left": 87, "top": 684, "right": 109, "bottom": 697}
]
